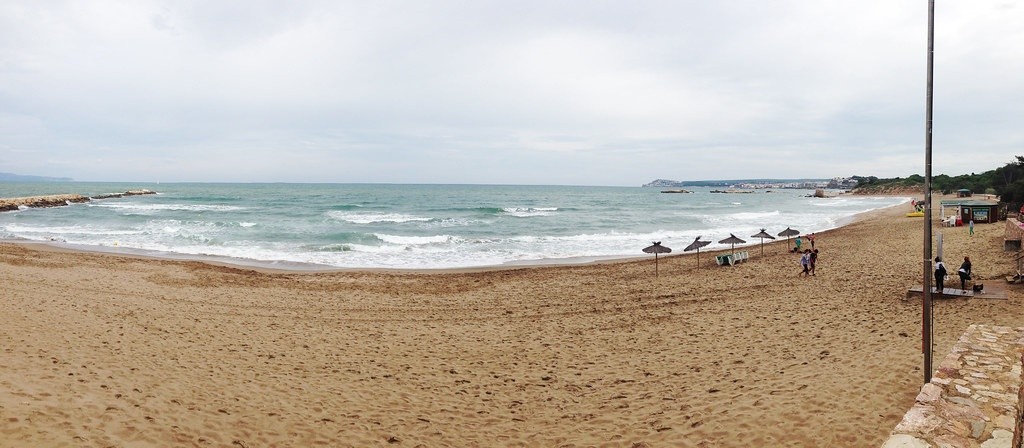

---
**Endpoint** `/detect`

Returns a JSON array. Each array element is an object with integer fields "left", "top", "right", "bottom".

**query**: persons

[
  {"left": 969, "top": 219, "right": 974, "bottom": 236},
  {"left": 796, "top": 233, "right": 818, "bottom": 278},
  {"left": 959, "top": 256, "right": 971, "bottom": 293},
  {"left": 934, "top": 256, "right": 947, "bottom": 293}
]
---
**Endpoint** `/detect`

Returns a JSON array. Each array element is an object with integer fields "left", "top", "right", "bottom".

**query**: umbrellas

[{"left": 643, "top": 227, "right": 800, "bottom": 274}]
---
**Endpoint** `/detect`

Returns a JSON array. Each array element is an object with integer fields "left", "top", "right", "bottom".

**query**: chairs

[
  {"left": 716, "top": 252, "right": 749, "bottom": 266},
  {"left": 943, "top": 216, "right": 964, "bottom": 227}
]
